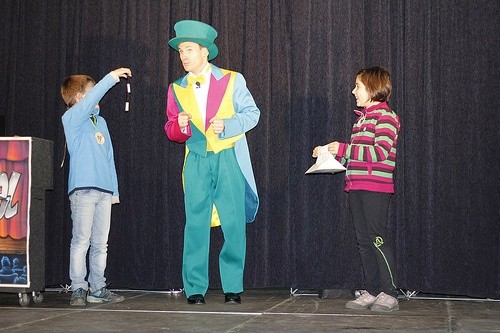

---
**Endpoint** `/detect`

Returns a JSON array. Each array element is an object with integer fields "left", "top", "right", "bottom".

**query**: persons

[
  {"left": 164, "top": 19, "right": 261, "bottom": 305},
  {"left": 61, "top": 67, "right": 132, "bottom": 307},
  {"left": 312, "top": 66, "right": 401, "bottom": 313}
]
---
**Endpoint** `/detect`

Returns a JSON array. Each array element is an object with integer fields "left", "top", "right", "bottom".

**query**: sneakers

[
  {"left": 70, "top": 288, "right": 88, "bottom": 306},
  {"left": 87, "top": 289, "right": 125, "bottom": 303},
  {"left": 370, "top": 293, "right": 400, "bottom": 313},
  {"left": 345, "top": 293, "right": 376, "bottom": 310}
]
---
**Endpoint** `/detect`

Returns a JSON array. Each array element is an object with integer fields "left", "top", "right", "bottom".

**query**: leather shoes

[
  {"left": 224, "top": 292, "right": 241, "bottom": 305},
  {"left": 187, "top": 294, "right": 205, "bottom": 304}
]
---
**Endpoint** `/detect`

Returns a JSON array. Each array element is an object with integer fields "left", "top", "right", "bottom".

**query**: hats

[{"left": 167, "top": 20, "right": 219, "bottom": 61}]
